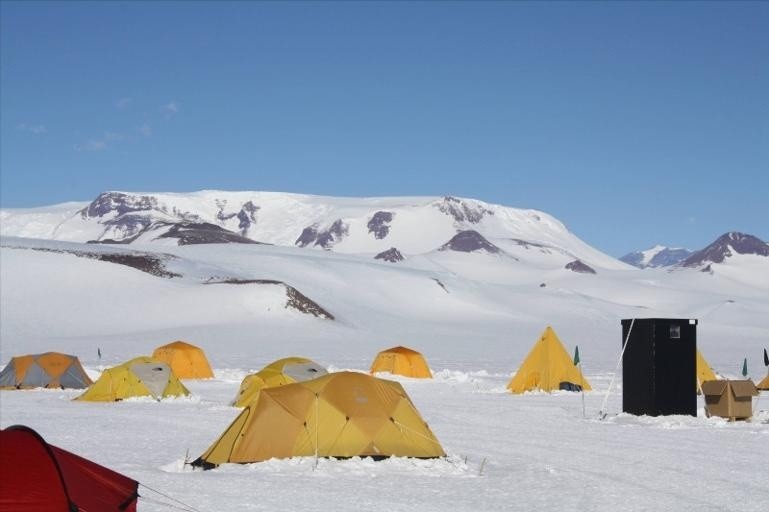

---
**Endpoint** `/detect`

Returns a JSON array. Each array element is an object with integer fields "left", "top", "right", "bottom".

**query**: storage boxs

[{"left": 698, "top": 380, "right": 759, "bottom": 418}]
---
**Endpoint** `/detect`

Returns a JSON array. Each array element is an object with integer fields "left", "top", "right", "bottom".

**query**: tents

[
  {"left": 231, "top": 357, "right": 329, "bottom": 408},
  {"left": 0, "top": 352, "right": 95, "bottom": 391},
  {"left": 0, "top": 425, "right": 140, "bottom": 511},
  {"left": 73, "top": 356, "right": 191, "bottom": 402},
  {"left": 507, "top": 326, "right": 594, "bottom": 394},
  {"left": 153, "top": 340, "right": 215, "bottom": 379},
  {"left": 369, "top": 346, "right": 434, "bottom": 379},
  {"left": 187, "top": 371, "right": 447, "bottom": 471}
]
